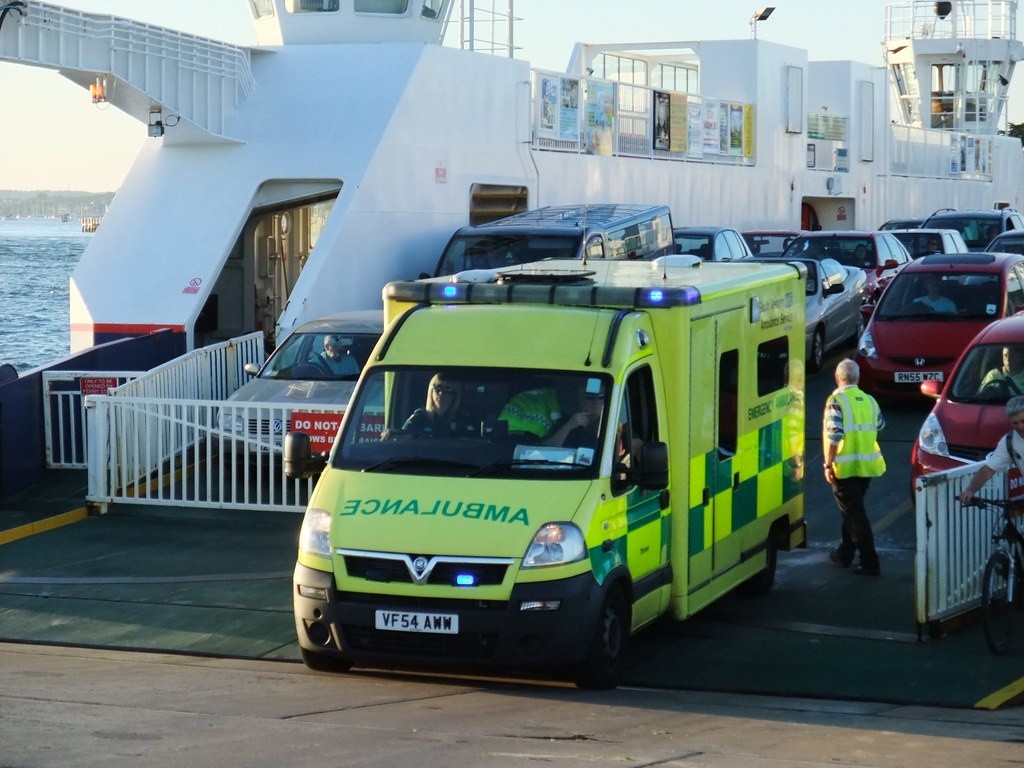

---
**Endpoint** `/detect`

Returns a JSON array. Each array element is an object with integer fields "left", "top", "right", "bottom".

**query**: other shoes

[
  {"left": 849, "top": 563, "right": 876, "bottom": 576},
  {"left": 830, "top": 550, "right": 850, "bottom": 568}
]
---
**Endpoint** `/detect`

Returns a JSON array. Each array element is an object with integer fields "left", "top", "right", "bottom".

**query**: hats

[{"left": 324, "top": 335, "right": 344, "bottom": 346}]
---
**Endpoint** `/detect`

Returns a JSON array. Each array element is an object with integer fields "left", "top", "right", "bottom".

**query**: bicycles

[{"left": 953, "top": 493, "right": 1023, "bottom": 655}]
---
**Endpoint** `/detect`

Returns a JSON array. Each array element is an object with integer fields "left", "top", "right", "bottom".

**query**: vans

[{"left": 418, "top": 203, "right": 675, "bottom": 281}]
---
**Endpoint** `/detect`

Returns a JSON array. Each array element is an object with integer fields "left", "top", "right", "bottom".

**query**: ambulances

[{"left": 283, "top": 254, "right": 810, "bottom": 693}]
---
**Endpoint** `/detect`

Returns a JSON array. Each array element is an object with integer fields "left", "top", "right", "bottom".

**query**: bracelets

[
  {"left": 823, "top": 463, "right": 832, "bottom": 468},
  {"left": 791, "top": 463, "right": 802, "bottom": 468}
]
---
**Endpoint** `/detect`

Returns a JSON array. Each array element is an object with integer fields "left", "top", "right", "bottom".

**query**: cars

[
  {"left": 905, "top": 312, "right": 1024, "bottom": 506},
  {"left": 734, "top": 253, "right": 869, "bottom": 372},
  {"left": 741, "top": 230, "right": 828, "bottom": 257},
  {"left": 673, "top": 228, "right": 752, "bottom": 264},
  {"left": 849, "top": 252, "right": 1024, "bottom": 403},
  {"left": 780, "top": 230, "right": 915, "bottom": 298},
  {"left": 877, "top": 207, "right": 1024, "bottom": 254},
  {"left": 214, "top": 310, "right": 392, "bottom": 480}
]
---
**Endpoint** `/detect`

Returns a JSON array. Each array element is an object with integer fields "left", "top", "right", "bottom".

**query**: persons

[
  {"left": 912, "top": 276, "right": 958, "bottom": 316},
  {"left": 760, "top": 360, "right": 805, "bottom": 501},
  {"left": 985, "top": 226, "right": 998, "bottom": 243},
  {"left": 926, "top": 238, "right": 941, "bottom": 255},
  {"left": 959, "top": 395, "right": 1024, "bottom": 518},
  {"left": 822, "top": 357, "right": 886, "bottom": 575},
  {"left": 976, "top": 345, "right": 1024, "bottom": 396},
  {"left": 540, "top": 380, "right": 630, "bottom": 481},
  {"left": 380, "top": 370, "right": 477, "bottom": 441},
  {"left": 306, "top": 334, "right": 361, "bottom": 376},
  {"left": 851, "top": 243, "right": 871, "bottom": 267}
]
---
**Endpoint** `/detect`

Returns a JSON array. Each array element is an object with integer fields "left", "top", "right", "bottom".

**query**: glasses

[
  {"left": 433, "top": 384, "right": 455, "bottom": 395},
  {"left": 584, "top": 392, "right": 606, "bottom": 400}
]
